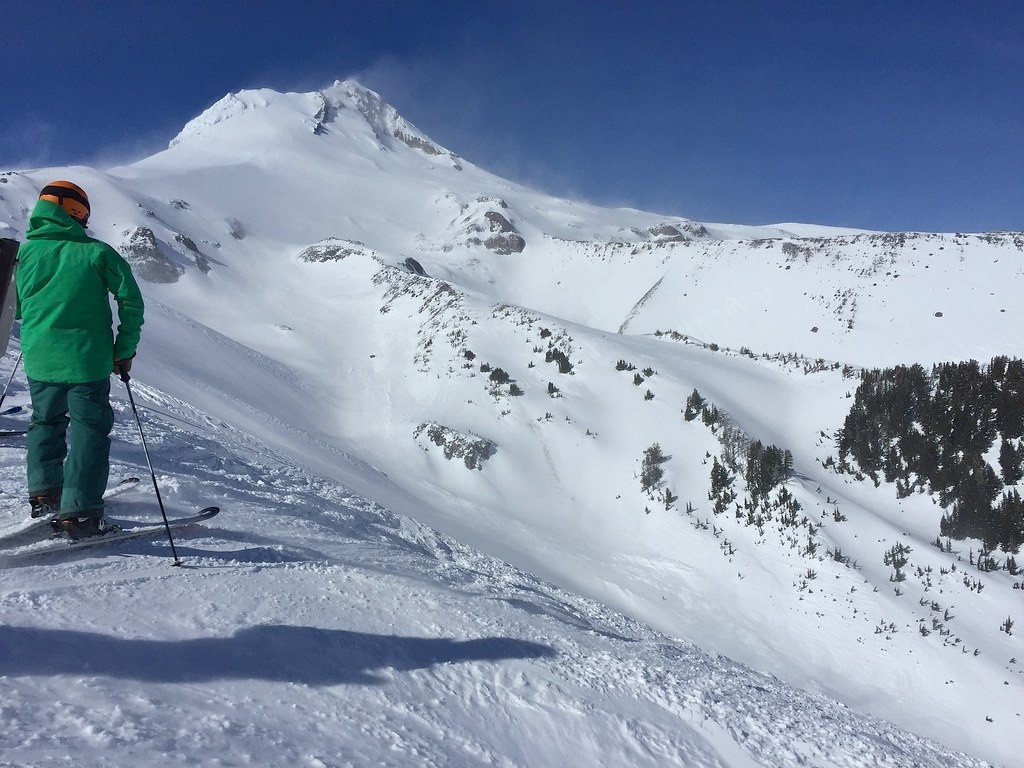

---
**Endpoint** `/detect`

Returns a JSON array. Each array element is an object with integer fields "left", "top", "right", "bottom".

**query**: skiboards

[
  {"left": 0, "top": 405, "right": 28, "bottom": 436},
  {"left": 1, "top": 476, "right": 222, "bottom": 563}
]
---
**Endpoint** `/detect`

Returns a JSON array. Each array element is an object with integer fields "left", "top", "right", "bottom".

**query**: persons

[{"left": 13, "top": 180, "right": 144, "bottom": 540}]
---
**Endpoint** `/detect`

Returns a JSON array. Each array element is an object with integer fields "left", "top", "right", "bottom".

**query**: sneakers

[
  {"left": 62, "top": 518, "right": 122, "bottom": 540},
  {"left": 29, "top": 495, "right": 60, "bottom": 518}
]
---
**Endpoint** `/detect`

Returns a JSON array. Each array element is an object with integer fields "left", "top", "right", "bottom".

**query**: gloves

[{"left": 113, "top": 350, "right": 136, "bottom": 373}]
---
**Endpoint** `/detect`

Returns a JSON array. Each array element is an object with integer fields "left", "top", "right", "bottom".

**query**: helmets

[{"left": 39, "top": 181, "right": 90, "bottom": 230}]
